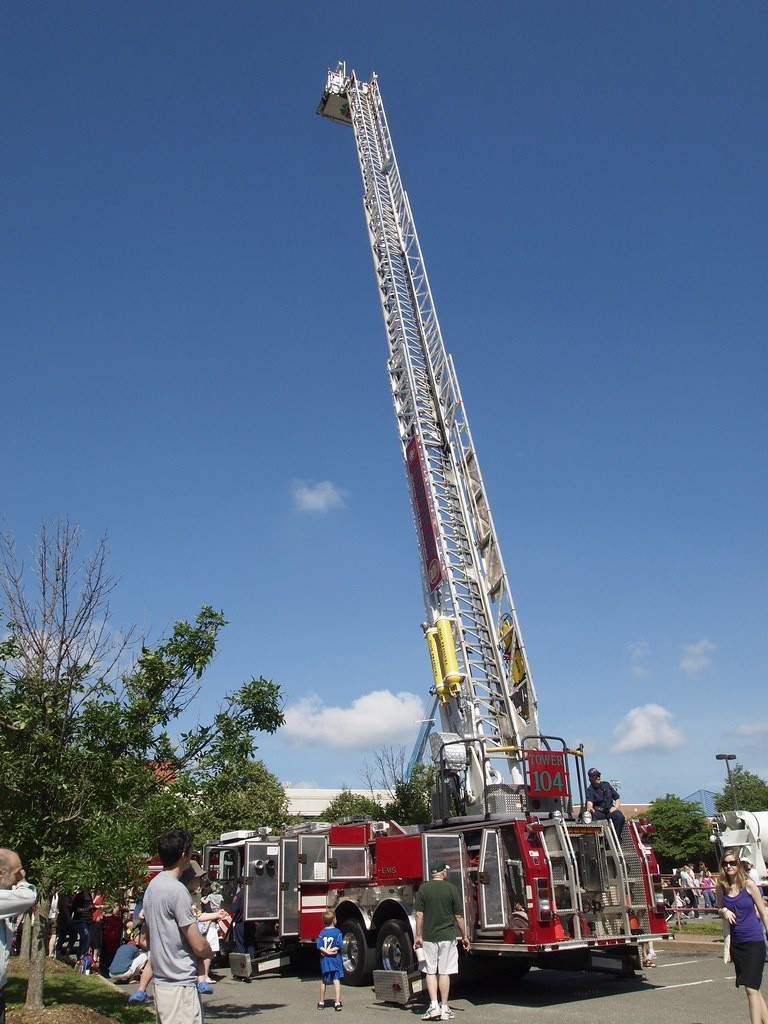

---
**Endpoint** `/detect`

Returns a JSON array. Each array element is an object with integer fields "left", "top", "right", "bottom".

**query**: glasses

[
  {"left": 593, "top": 775, "right": 600, "bottom": 778},
  {"left": 722, "top": 861, "right": 737, "bottom": 867}
]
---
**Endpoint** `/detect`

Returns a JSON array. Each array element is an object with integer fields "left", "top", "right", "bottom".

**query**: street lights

[{"left": 715, "top": 753, "right": 739, "bottom": 812}]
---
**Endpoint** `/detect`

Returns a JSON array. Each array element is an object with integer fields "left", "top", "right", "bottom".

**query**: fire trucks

[{"left": 133, "top": 59, "right": 676, "bottom": 1006}]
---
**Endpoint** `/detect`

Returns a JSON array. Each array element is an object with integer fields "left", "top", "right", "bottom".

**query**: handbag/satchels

[
  {"left": 74, "top": 953, "right": 92, "bottom": 976},
  {"left": 723, "top": 918, "right": 732, "bottom": 964}
]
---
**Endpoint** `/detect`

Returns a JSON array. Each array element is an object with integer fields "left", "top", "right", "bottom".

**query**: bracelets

[
  {"left": 15, "top": 925, "right": 19, "bottom": 927},
  {"left": 84, "top": 907, "right": 88, "bottom": 911},
  {"left": 80, "top": 908, "right": 82, "bottom": 912},
  {"left": 721, "top": 907, "right": 728, "bottom": 916}
]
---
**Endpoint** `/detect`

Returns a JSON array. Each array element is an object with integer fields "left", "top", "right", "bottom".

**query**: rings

[{"left": 91, "top": 906, "right": 92, "bottom": 907}]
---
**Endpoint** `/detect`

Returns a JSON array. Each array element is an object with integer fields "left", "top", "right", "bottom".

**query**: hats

[
  {"left": 588, "top": 768, "right": 601, "bottom": 777},
  {"left": 180, "top": 860, "right": 207, "bottom": 882},
  {"left": 127, "top": 941, "right": 136, "bottom": 946},
  {"left": 431, "top": 860, "right": 450, "bottom": 874},
  {"left": 211, "top": 881, "right": 223, "bottom": 891},
  {"left": 237, "top": 876, "right": 244, "bottom": 883},
  {"left": 688, "top": 863, "right": 695, "bottom": 867}
]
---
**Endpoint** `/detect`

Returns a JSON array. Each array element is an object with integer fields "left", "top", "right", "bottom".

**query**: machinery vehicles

[{"left": 708, "top": 808, "right": 768, "bottom": 887}]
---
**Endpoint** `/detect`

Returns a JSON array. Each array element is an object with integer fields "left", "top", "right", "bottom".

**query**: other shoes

[
  {"left": 206, "top": 977, "right": 217, "bottom": 984},
  {"left": 334, "top": 1003, "right": 342, "bottom": 1010},
  {"left": 695, "top": 916, "right": 703, "bottom": 919},
  {"left": 646, "top": 951, "right": 658, "bottom": 960},
  {"left": 317, "top": 1001, "right": 324, "bottom": 1010},
  {"left": 127, "top": 980, "right": 139, "bottom": 984}
]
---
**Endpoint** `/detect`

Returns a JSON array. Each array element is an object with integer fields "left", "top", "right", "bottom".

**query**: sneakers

[
  {"left": 440, "top": 1007, "right": 454, "bottom": 1020},
  {"left": 421, "top": 1004, "right": 442, "bottom": 1020}
]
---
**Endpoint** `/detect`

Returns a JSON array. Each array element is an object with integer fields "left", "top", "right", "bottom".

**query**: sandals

[
  {"left": 643, "top": 959, "right": 657, "bottom": 968},
  {"left": 129, "top": 991, "right": 149, "bottom": 1003},
  {"left": 196, "top": 981, "right": 213, "bottom": 994}
]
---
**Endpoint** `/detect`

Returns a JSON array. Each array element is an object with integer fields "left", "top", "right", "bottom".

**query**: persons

[
  {"left": 197, "top": 877, "right": 224, "bottom": 983},
  {"left": 579, "top": 768, "right": 625, "bottom": 849},
  {"left": 128, "top": 860, "right": 229, "bottom": 1002},
  {"left": 715, "top": 849, "right": 768, "bottom": 1024},
  {"left": 0, "top": 848, "right": 37, "bottom": 1024},
  {"left": 740, "top": 857, "right": 768, "bottom": 961},
  {"left": 232, "top": 875, "right": 259, "bottom": 983},
  {"left": 639, "top": 941, "right": 657, "bottom": 968},
  {"left": 317, "top": 911, "right": 344, "bottom": 1011},
  {"left": 14, "top": 887, "right": 147, "bottom": 984},
  {"left": 413, "top": 860, "right": 471, "bottom": 1021},
  {"left": 143, "top": 828, "right": 212, "bottom": 1024},
  {"left": 661, "top": 861, "right": 718, "bottom": 919}
]
